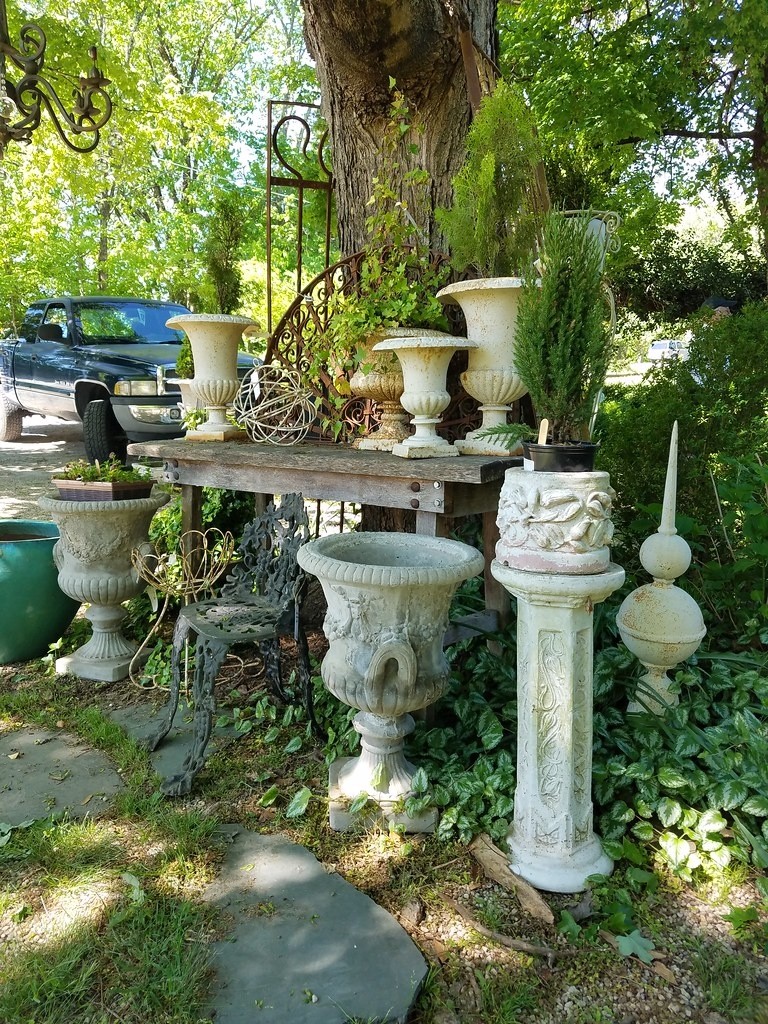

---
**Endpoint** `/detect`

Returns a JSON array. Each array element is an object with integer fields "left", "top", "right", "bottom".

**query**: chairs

[{"left": 134, "top": 492, "right": 334, "bottom": 795}]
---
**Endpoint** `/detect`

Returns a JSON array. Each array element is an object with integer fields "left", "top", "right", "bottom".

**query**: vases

[{"left": 371, "top": 337, "right": 478, "bottom": 459}]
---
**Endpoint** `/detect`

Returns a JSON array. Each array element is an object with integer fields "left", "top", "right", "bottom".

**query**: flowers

[{"left": 51, "top": 451, "right": 157, "bottom": 482}]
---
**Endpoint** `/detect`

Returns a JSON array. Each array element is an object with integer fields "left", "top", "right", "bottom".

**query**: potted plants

[
  {"left": 166, "top": 333, "right": 207, "bottom": 428},
  {"left": 434, "top": 79, "right": 550, "bottom": 456},
  {"left": 474, "top": 205, "right": 624, "bottom": 473},
  {"left": 299, "top": 76, "right": 453, "bottom": 452},
  {"left": 164, "top": 181, "right": 261, "bottom": 441}
]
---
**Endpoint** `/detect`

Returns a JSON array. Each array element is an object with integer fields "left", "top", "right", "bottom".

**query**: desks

[{"left": 126, "top": 439, "right": 523, "bottom": 741}]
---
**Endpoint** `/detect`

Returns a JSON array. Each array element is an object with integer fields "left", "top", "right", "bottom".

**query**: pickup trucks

[{"left": 0, "top": 295, "right": 264, "bottom": 464}]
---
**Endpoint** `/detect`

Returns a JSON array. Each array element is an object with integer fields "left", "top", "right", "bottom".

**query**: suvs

[{"left": 647, "top": 340, "right": 687, "bottom": 365}]
